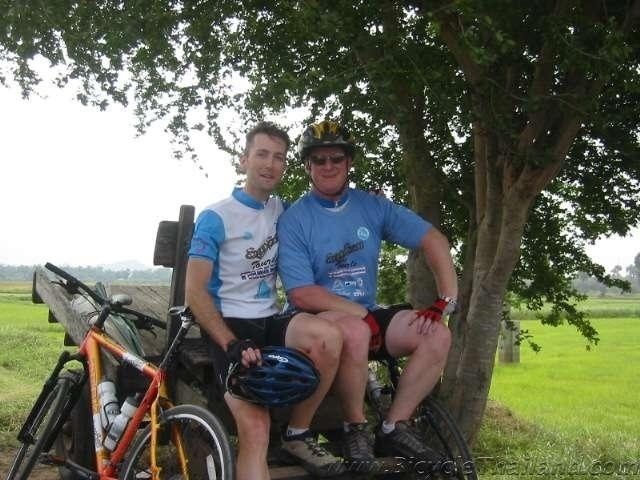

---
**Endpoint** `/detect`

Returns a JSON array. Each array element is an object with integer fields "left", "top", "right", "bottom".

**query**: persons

[
  {"left": 274, "top": 119, "right": 460, "bottom": 465},
  {"left": 183, "top": 121, "right": 391, "bottom": 479}
]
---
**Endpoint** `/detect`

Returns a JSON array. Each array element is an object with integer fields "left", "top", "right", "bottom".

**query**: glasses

[{"left": 306, "top": 152, "right": 348, "bottom": 165}]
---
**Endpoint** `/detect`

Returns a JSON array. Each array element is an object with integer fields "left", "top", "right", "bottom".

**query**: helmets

[
  {"left": 297, "top": 120, "right": 356, "bottom": 164},
  {"left": 224, "top": 346, "right": 320, "bottom": 409}
]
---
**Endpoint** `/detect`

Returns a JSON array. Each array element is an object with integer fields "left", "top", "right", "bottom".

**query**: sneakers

[
  {"left": 343, "top": 421, "right": 377, "bottom": 463},
  {"left": 277, "top": 428, "right": 338, "bottom": 470},
  {"left": 377, "top": 419, "right": 435, "bottom": 458}
]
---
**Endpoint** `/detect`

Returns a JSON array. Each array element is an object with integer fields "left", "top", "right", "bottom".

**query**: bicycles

[{"left": 8, "top": 263, "right": 236, "bottom": 480}]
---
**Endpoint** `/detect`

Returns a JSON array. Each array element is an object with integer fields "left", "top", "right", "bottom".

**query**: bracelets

[{"left": 436, "top": 295, "right": 458, "bottom": 307}]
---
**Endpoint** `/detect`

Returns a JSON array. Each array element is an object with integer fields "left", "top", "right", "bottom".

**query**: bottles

[
  {"left": 103, "top": 393, "right": 142, "bottom": 451},
  {"left": 367, "top": 368, "right": 381, "bottom": 401},
  {"left": 97, "top": 375, "right": 120, "bottom": 428}
]
